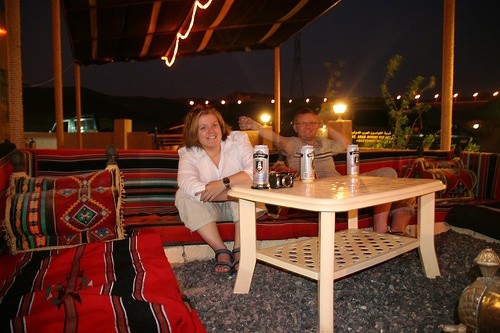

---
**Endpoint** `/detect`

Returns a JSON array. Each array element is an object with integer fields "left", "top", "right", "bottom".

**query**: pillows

[
  {"left": 0, "top": 165, "right": 126, "bottom": 256},
  {"left": 411, "top": 157, "right": 477, "bottom": 203}
]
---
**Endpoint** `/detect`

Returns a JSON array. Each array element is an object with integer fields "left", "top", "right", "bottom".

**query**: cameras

[{"left": 269, "top": 171, "right": 293, "bottom": 189}]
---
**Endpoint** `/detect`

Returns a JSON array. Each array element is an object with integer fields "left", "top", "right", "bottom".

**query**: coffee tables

[{"left": 226, "top": 174, "right": 448, "bottom": 333}]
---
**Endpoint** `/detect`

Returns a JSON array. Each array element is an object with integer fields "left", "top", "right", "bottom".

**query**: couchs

[{"left": 0, "top": 148, "right": 500, "bottom": 333}]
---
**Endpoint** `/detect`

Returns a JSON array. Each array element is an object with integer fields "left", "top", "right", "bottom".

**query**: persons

[
  {"left": 238, "top": 108, "right": 416, "bottom": 238},
  {"left": 174, "top": 104, "right": 254, "bottom": 276}
]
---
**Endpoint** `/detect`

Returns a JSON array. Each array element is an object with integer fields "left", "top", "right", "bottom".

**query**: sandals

[
  {"left": 211, "top": 249, "right": 232, "bottom": 276},
  {"left": 231, "top": 246, "right": 241, "bottom": 273}
]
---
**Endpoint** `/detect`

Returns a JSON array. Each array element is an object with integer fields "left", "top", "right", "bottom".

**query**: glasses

[{"left": 295, "top": 120, "right": 319, "bottom": 127}]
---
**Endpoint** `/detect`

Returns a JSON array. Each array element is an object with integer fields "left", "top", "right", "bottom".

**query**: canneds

[
  {"left": 347, "top": 144, "right": 359, "bottom": 176},
  {"left": 252, "top": 145, "right": 269, "bottom": 185},
  {"left": 301, "top": 145, "right": 314, "bottom": 182}
]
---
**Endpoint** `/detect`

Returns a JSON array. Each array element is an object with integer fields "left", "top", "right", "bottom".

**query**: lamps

[
  {"left": 327, "top": 99, "right": 352, "bottom": 146},
  {"left": 259, "top": 113, "right": 274, "bottom": 151}
]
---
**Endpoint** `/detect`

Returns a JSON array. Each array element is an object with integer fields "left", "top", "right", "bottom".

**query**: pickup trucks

[{"left": 49, "top": 113, "right": 114, "bottom": 134}]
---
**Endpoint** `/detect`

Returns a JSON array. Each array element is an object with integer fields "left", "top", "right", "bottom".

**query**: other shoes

[{"left": 386, "top": 230, "right": 416, "bottom": 257}]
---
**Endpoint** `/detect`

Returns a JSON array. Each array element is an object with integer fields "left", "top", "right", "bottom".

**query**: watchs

[{"left": 223, "top": 177, "right": 230, "bottom": 188}]
